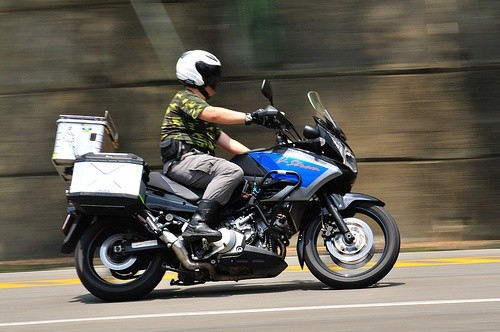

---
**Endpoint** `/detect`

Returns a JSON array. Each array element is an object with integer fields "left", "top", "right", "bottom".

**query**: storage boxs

[
  {"left": 69, "top": 151, "right": 151, "bottom": 216},
  {"left": 51, "top": 111, "right": 119, "bottom": 181}
]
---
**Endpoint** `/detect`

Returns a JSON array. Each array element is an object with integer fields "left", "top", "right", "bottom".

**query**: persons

[{"left": 159, "top": 49, "right": 285, "bottom": 243}]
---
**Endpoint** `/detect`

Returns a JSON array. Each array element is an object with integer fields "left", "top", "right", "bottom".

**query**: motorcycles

[{"left": 51, "top": 78, "right": 403, "bottom": 293}]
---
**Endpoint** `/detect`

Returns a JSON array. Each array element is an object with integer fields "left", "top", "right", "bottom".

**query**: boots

[{"left": 182, "top": 199, "right": 224, "bottom": 243}]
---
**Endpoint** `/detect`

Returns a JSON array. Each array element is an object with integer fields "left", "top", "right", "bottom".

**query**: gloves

[{"left": 251, "top": 105, "right": 285, "bottom": 129}]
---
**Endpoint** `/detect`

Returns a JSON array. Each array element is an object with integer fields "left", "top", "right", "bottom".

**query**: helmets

[{"left": 175, "top": 49, "right": 221, "bottom": 87}]
